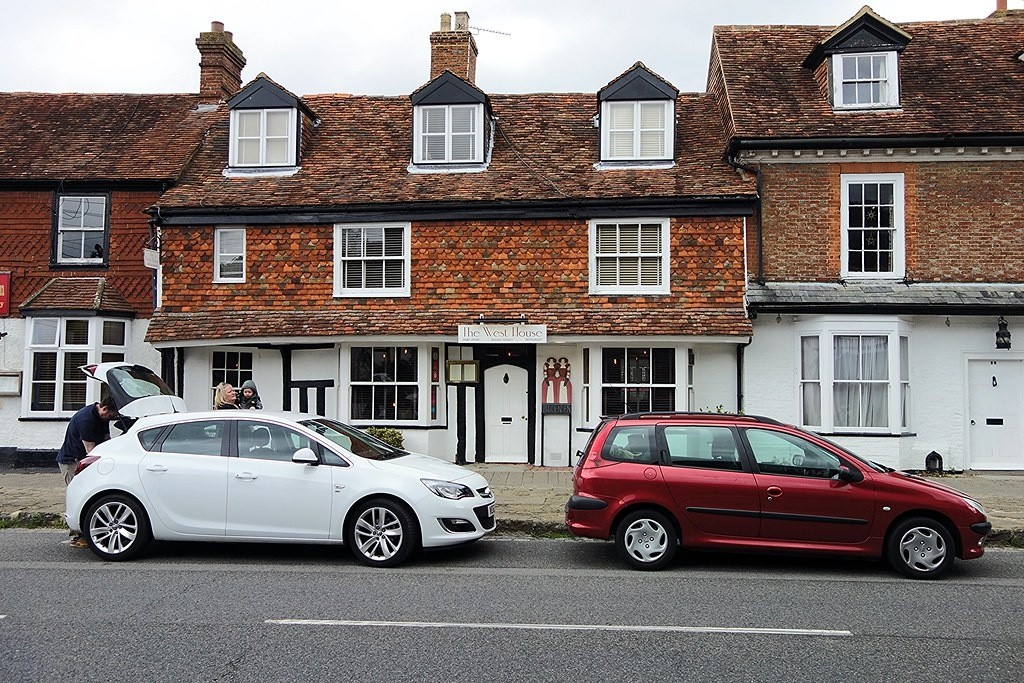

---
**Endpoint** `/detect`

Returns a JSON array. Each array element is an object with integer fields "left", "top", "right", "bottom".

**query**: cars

[{"left": 64, "top": 361, "right": 497, "bottom": 568}]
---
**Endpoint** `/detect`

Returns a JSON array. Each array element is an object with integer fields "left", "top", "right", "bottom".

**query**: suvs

[{"left": 565, "top": 412, "right": 992, "bottom": 580}]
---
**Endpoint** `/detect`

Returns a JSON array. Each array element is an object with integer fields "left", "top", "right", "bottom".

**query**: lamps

[
  {"left": 776, "top": 314, "right": 781, "bottom": 323},
  {"left": 945, "top": 317, "right": 951, "bottom": 327},
  {"left": 995, "top": 316, "right": 1012, "bottom": 350}
]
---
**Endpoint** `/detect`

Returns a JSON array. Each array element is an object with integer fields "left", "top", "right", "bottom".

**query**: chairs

[
  {"left": 250, "top": 428, "right": 277, "bottom": 459},
  {"left": 711, "top": 434, "right": 737, "bottom": 462}
]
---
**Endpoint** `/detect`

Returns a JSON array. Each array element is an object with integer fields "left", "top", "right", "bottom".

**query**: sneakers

[{"left": 69, "top": 535, "right": 90, "bottom": 548}]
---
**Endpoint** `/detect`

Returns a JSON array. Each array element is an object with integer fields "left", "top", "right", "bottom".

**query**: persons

[
  {"left": 214, "top": 380, "right": 263, "bottom": 454},
  {"left": 56, "top": 396, "right": 119, "bottom": 548}
]
